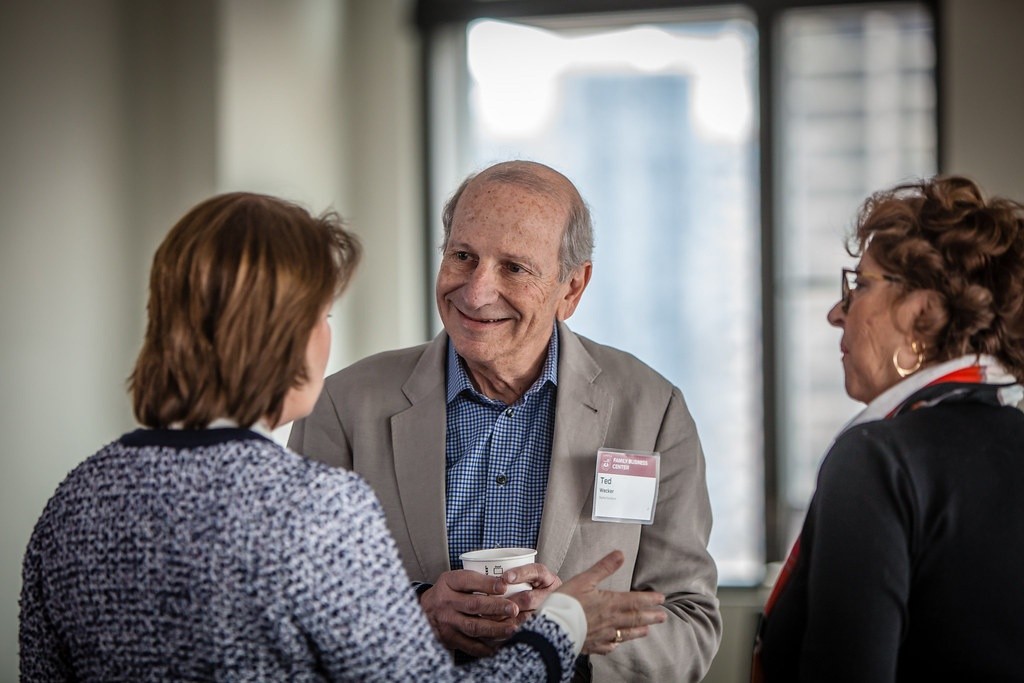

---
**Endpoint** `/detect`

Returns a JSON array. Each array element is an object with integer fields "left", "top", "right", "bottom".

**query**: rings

[{"left": 615, "top": 629, "right": 623, "bottom": 643}]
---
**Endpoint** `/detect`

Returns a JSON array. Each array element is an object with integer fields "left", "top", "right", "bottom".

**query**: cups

[{"left": 460, "top": 546, "right": 540, "bottom": 641}]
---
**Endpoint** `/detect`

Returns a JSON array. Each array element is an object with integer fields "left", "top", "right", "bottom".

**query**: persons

[
  {"left": 752, "top": 177, "right": 1024, "bottom": 683},
  {"left": 288, "top": 158, "right": 723, "bottom": 681},
  {"left": 17, "top": 192, "right": 668, "bottom": 682}
]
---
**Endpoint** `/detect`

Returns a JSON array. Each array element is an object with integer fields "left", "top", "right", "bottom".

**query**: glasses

[{"left": 842, "top": 267, "right": 915, "bottom": 306}]
